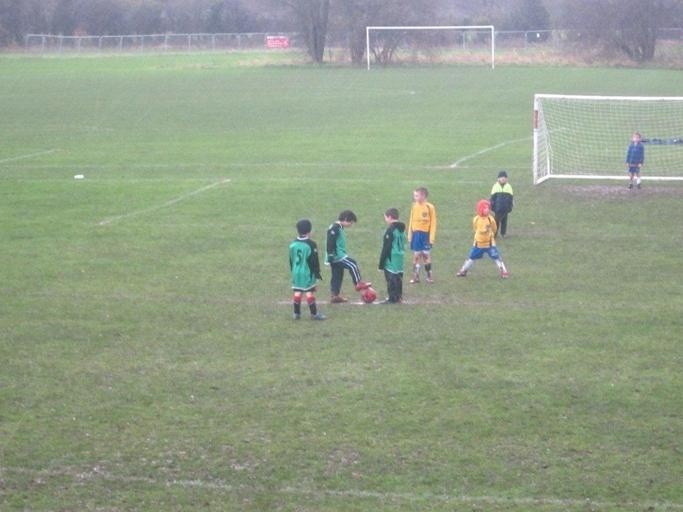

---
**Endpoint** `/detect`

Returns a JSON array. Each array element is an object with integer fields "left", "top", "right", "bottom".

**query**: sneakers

[
  {"left": 356, "top": 281, "right": 372, "bottom": 292},
  {"left": 410, "top": 270, "right": 510, "bottom": 283},
  {"left": 331, "top": 294, "right": 349, "bottom": 304},
  {"left": 310, "top": 311, "right": 329, "bottom": 320},
  {"left": 380, "top": 296, "right": 404, "bottom": 304},
  {"left": 292, "top": 312, "right": 300, "bottom": 321}
]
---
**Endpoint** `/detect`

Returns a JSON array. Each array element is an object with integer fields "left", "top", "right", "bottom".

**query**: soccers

[{"left": 361, "top": 288, "right": 377, "bottom": 303}]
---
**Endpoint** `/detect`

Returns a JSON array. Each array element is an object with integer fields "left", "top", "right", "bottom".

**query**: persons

[
  {"left": 323, "top": 209, "right": 372, "bottom": 303},
  {"left": 455, "top": 200, "right": 508, "bottom": 278},
  {"left": 489, "top": 171, "right": 513, "bottom": 239},
  {"left": 407, "top": 188, "right": 436, "bottom": 283},
  {"left": 622, "top": 132, "right": 644, "bottom": 189},
  {"left": 287, "top": 219, "right": 328, "bottom": 321},
  {"left": 377, "top": 207, "right": 405, "bottom": 303}
]
